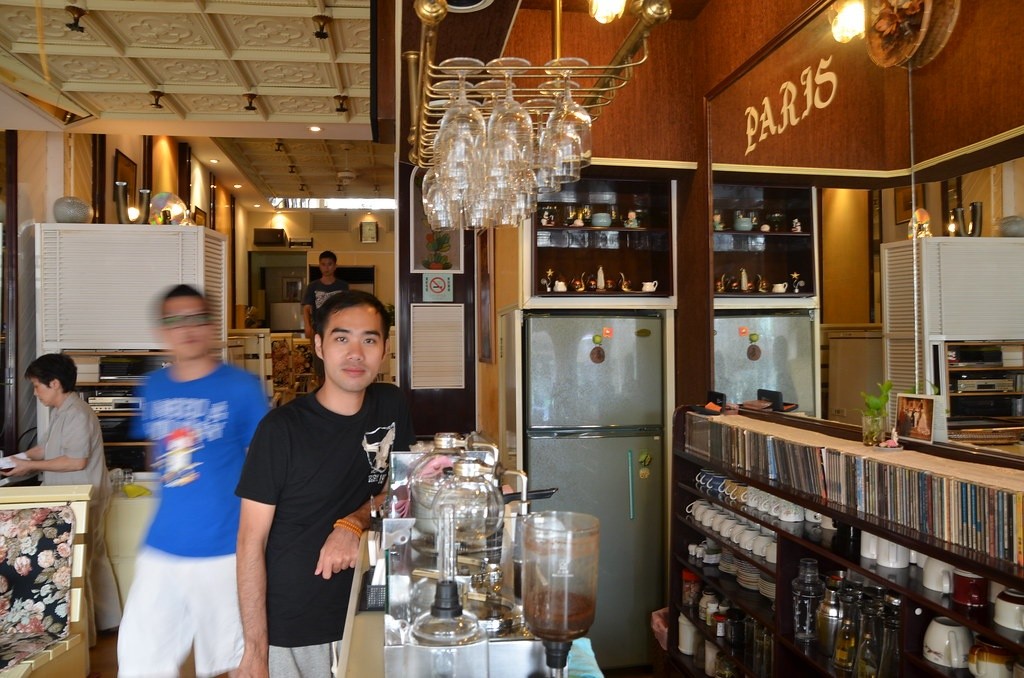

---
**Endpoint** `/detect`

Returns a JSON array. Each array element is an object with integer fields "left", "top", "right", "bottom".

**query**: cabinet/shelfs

[
  {"left": 666, "top": 403, "right": 1024, "bottom": 678},
  {"left": 61, "top": 346, "right": 180, "bottom": 472},
  {"left": 519, "top": 175, "right": 678, "bottom": 309},
  {"left": 943, "top": 341, "right": 1024, "bottom": 429},
  {"left": 713, "top": 180, "right": 818, "bottom": 301}
]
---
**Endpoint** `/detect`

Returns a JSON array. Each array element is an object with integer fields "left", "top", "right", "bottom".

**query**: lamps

[
  {"left": 825, "top": 0, "right": 864, "bottom": 43},
  {"left": 588, "top": 0, "right": 627, "bottom": 24}
]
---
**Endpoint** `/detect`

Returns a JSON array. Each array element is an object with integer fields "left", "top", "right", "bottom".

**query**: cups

[
  {"left": 771, "top": 282, "right": 788, "bottom": 294},
  {"left": 641, "top": 280, "right": 658, "bottom": 292},
  {"left": 685, "top": 467, "right": 838, "bottom": 564},
  {"left": 859, "top": 530, "right": 1024, "bottom": 678},
  {"left": 732, "top": 217, "right": 753, "bottom": 231},
  {"left": 591, "top": 212, "right": 612, "bottom": 227}
]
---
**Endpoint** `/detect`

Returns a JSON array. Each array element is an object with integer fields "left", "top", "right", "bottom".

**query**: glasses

[{"left": 154, "top": 312, "right": 212, "bottom": 330}]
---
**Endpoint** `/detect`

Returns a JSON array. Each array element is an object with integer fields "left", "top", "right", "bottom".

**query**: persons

[
  {"left": 234, "top": 289, "right": 418, "bottom": 678},
  {"left": 301, "top": 251, "right": 349, "bottom": 384},
  {"left": 899, "top": 407, "right": 931, "bottom": 437},
  {"left": 0, "top": 353, "right": 122, "bottom": 648},
  {"left": 117, "top": 283, "right": 272, "bottom": 678}
]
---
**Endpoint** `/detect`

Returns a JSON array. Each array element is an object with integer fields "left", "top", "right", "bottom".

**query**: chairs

[
  {"left": 226, "top": 328, "right": 314, "bottom": 404},
  {"left": 0, "top": 501, "right": 91, "bottom": 678}
]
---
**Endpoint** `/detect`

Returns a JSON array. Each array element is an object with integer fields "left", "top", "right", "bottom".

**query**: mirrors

[{"left": 704, "top": 0, "right": 1024, "bottom": 474}]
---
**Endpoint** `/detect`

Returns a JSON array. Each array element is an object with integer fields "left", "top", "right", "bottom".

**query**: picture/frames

[
  {"left": 894, "top": 185, "right": 927, "bottom": 225},
  {"left": 113, "top": 148, "right": 137, "bottom": 208},
  {"left": 475, "top": 224, "right": 496, "bottom": 365},
  {"left": 282, "top": 276, "right": 302, "bottom": 303},
  {"left": 360, "top": 221, "right": 378, "bottom": 244},
  {"left": 195, "top": 205, "right": 207, "bottom": 226}
]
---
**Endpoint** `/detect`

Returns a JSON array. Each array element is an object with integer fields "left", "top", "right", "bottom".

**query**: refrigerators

[
  {"left": 523, "top": 313, "right": 667, "bottom": 671},
  {"left": 711, "top": 314, "right": 820, "bottom": 419}
]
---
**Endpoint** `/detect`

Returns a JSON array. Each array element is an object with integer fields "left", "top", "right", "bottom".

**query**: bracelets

[{"left": 334, "top": 519, "right": 362, "bottom": 537}]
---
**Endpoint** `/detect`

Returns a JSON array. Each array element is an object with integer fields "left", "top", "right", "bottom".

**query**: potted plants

[{"left": 859, "top": 378, "right": 894, "bottom": 448}]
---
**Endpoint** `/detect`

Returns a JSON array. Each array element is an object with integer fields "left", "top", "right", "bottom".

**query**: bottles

[
  {"left": 790, "top": 556, "right": 903, "bottom": 678},
  {"left": 678, "top": 568, "right": 772, "bottom": 678}
]
